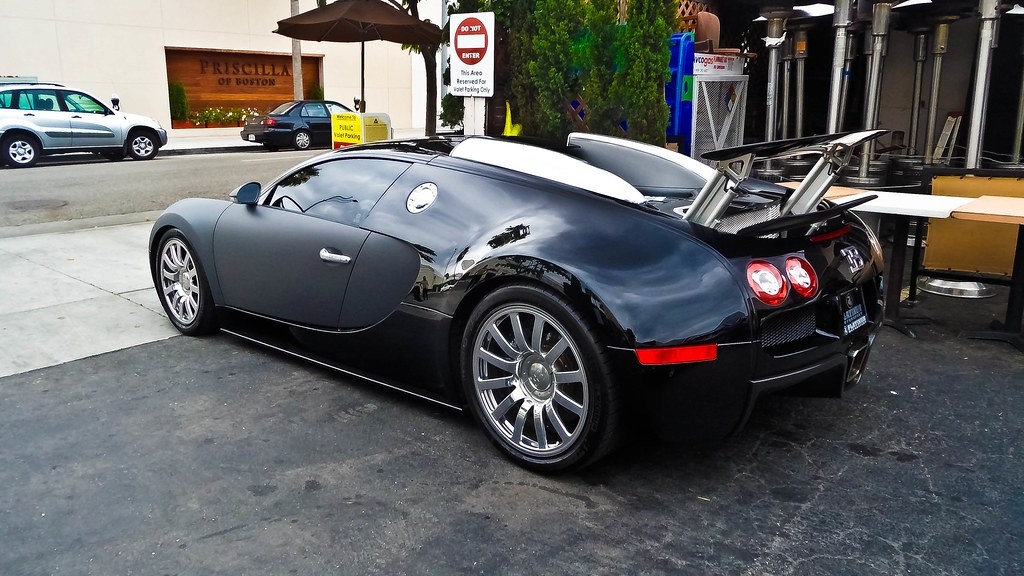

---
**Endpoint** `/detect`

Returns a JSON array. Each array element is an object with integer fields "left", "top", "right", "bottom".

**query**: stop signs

[{"left": 453, "top": 16, "right": 489, "bottom": 66}]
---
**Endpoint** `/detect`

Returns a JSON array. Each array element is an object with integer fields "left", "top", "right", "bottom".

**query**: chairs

[
  {"left": 45, "top": 98, "right": 53, "bottom": 111},
  {"left": 38, "top": 99, "right": 45, "bottom": 110}
]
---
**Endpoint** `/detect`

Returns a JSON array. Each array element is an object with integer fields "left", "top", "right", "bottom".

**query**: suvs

[{"left": 0, "top": 76, "right": 169, "bottom": 169}]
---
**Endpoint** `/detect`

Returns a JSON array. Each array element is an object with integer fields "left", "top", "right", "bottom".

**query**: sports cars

[{"left": 149, "top": 128, "right": 889, "bottom": 475}]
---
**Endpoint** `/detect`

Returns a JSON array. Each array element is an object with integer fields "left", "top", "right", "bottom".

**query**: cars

[{"left": 239, "top": 99, "right": 356, "bottom": 149}]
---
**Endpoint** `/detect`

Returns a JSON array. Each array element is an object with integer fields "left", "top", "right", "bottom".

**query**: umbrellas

[{"left": 272, "top": 0, "right": 442, "bottom": 113}]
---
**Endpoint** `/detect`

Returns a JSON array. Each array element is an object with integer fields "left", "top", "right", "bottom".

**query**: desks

[{"left": 776, "top": 182, "right": 1024, "bottom": 353}]
